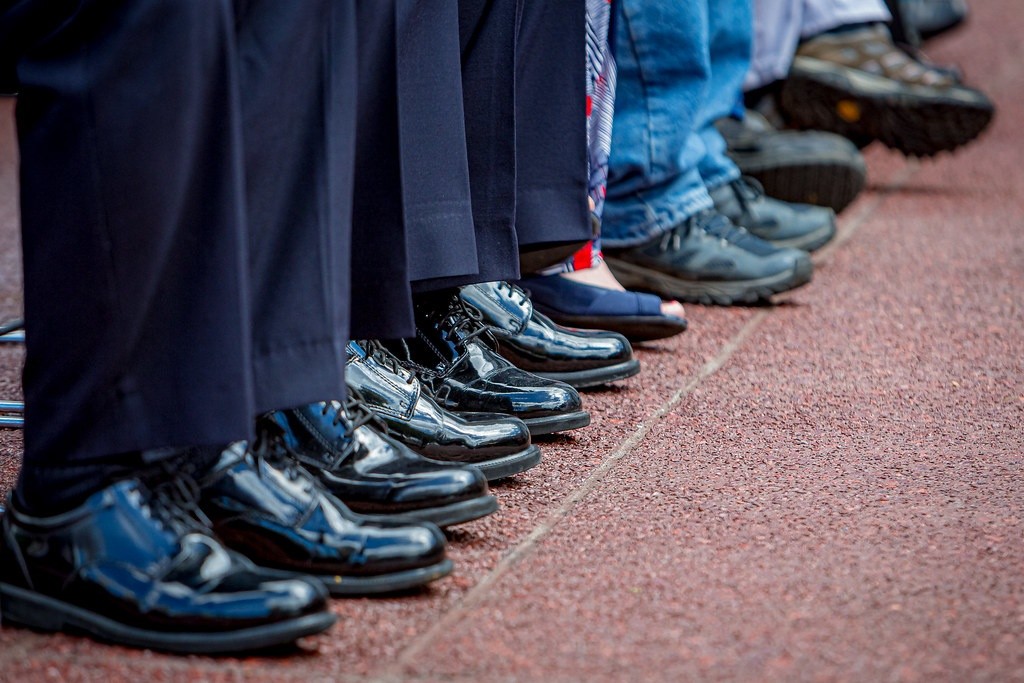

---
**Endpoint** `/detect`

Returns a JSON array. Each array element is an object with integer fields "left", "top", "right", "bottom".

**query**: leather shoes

[
  {"left": 0, "top": 476, "right": 338, "bottom": 652},
  {"left": 261, "top": 382, "right": 498, "bottom": 527},
  {"left": 197, "top": 436, "right": 455, "bottom": 594},
  {"left": 342, "top": 334, "right": 541, "bottom": 482},
  {"left": 455, "top": 279, "right": 642, "bottom": 387},
  {"left": 401, "top": 287, "right": 590, "bottom": 438}
]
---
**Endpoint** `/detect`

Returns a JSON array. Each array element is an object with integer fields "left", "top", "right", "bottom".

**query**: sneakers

[
  {"left": 718, "top": 112, "right": 864, "bottom": 215},
  {"left": 710, "top": 179, "right": 835, "bottom": 256},
  {"left": 776, "top": 23, "right": 996, "bottom": 158},
  {"left": 602, "top": 205, "right": 816, "bottom": 308}
]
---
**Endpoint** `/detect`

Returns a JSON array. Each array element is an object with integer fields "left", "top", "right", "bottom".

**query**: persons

[{"left": 0, "top": 0, "right": 997, "bottom": 652}]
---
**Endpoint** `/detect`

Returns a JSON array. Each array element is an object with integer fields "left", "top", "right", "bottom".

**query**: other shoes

[{"left": 514, "top": 270, "right": 687, "bottom": 340}]
